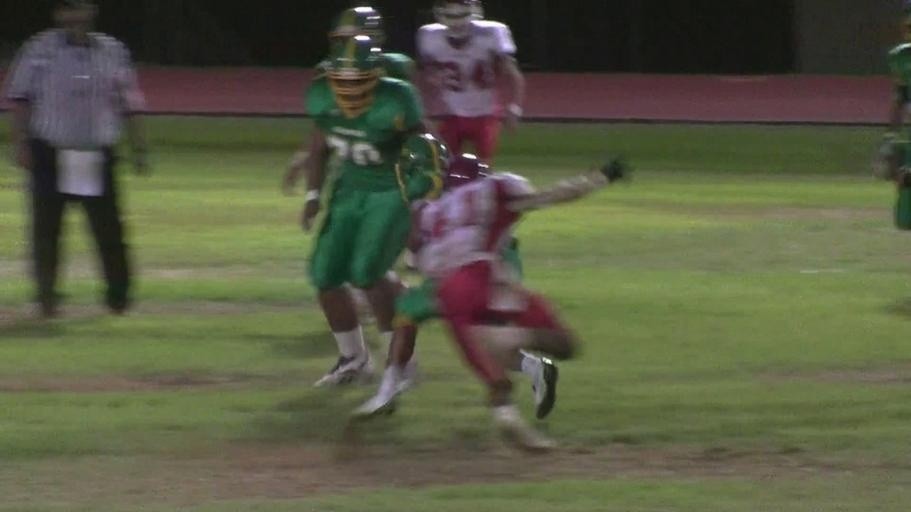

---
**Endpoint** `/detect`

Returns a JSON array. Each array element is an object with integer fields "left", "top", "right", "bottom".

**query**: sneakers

[
  {"left": 532, "top": 355, "right": 559, "bottom": 418},
  {"left": 312, "top": 351, "right": 374, "bottom": 389},
  {"left": 492, "top": 403, "right": 556, "bottom": 453},
  {"left": 351, "top": 370, "right": 417, "bottom": 418}
]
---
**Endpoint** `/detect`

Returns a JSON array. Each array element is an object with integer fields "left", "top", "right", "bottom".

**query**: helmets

[
  {"left": 431, "top": 0, "right": 483, "bottom": 43},
  {"left": 393, "top": 133, "right": 490, "bottom": 193},
  {"left": 322, "top": 6, "right": 384, "bottom": 119}
]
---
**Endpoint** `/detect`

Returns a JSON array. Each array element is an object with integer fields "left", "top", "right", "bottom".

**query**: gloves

[{"left": 602, "top": 153, "right": 627, "bottom": 182}]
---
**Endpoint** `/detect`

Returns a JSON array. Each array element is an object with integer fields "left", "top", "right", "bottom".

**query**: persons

[
  {"left": 876, "top": 41, "right": 911, "bottom": 232},
  {"left": 1, "top": 0, "right": 150, "bottom": 314}
]
